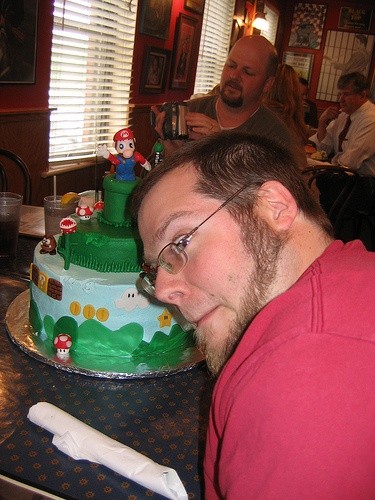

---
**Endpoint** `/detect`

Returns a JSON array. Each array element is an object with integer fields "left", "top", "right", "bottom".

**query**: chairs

[
  {"left": 301, "top": 160, "right": 375, "bottom": 251},
  {"left": 0, "top": 150, "right": 31, "bottom": 204}
]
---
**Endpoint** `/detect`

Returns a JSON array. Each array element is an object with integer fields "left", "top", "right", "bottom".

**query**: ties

[{"left": 337, "top": 116, "right": 352, "bottom": 152}]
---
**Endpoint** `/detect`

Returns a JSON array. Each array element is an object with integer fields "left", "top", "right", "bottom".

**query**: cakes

[{"left": 27, "top": 128, "right": 195, "bottom": 359}]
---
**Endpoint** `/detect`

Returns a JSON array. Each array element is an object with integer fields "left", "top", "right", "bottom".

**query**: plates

[{"left": 305, "top": 152, "right": 328, "bottom": 161}]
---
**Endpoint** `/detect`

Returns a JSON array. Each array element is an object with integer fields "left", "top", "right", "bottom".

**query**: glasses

[{"left": 141, "top": 181, "right": 264, "bottom": 284}]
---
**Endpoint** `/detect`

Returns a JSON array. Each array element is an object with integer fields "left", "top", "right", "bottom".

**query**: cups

[
  {"left": 0, "top": 192, "right": 23, "bottom": 258},
  {"left": 150, "top": 101, "right": 192, "bottom": 138},
  {"left": 44, "top": 196, "right": 79, "bottom": 236}
]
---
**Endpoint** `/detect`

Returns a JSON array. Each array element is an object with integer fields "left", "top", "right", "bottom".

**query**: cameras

[{"left": 159, "top": 101, "right": 192, "bottom": 141}]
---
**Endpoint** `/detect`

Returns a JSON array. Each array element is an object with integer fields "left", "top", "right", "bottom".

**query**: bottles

[{"left": 141, "top": 142, "right": 169, "bottom": 181}]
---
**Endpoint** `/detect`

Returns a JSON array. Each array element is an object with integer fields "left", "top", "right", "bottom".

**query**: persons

[
  {"left": 307, "top": 71, "right": 375, "bottom": 177},
  {"left": 298, "top": 76, "right": 318, "bottom": 130},
  {"left": 151, "top": 35, "right": 291, "bottom": 161},
  {"left": 262, "top": 63, "right": 309, "bottom": 156},
  {"left": 130, "top": 133, "right": 375, "bottom": 500}
]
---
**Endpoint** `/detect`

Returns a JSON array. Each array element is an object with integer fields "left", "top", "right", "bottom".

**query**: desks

[{"left": 0, "top": 205, "right": 216, "bottom": 500}]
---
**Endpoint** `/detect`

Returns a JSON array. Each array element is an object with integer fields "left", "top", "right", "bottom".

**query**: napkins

[{"left": 27, "top": 401, "right": 187, "bottom": 499}]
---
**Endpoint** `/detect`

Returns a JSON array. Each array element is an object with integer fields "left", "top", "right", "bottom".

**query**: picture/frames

[
  {"left": 170, "top": 12, "right": 198, "bottom": 91},
  {"left": 337, "top": 7, "right": 373, "bottom": 32},
  {"left": 140, "top": 0, "right": 174, "bottom": 39},
  {"left": 139, "top": 43, "right": 173, "bottom": 94},
  {"left": 184, "top": 0, "right": 205, "bottom": 15},
  {"left": 0, "top": 0, "right": 40, "bottom": 85},
  {"left": 282, "top": 51, "right": 315, "bottom": 96}
]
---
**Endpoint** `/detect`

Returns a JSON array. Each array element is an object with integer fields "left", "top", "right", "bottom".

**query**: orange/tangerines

[{"left": 61, "top": 192, "right": 81, "bottom": 205}]
